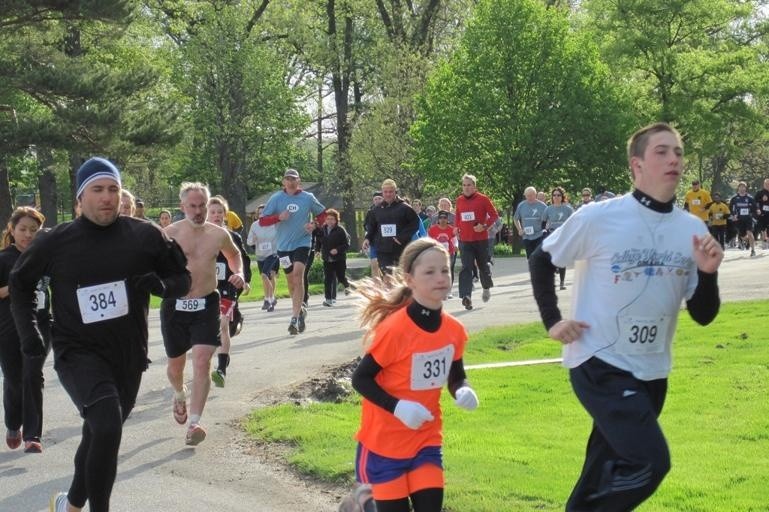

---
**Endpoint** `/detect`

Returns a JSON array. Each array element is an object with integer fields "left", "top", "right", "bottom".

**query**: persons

[
  {"left": 514, "top": 186, "right": 624, "bottom": 290},
  {"left": 362, "top": 172, "right": 502, "bottom": 309},
  {"left": 246, "top": 205, "right": 279, "bottom": 312},
  {"left": 7, "top": 156, "right": 194, "bottom": 512},
  {"left": 259, "top": 169, "right": 351, "bottom": 335},
  {"left": 73, "top": 187, "right": 253, "bottom": 389},
  {"left": 528, "top": 123, "right": 724, "bottom": 512},
  {"left": 159, "top": 182, "right": 246, "bottom": 446},
  {"left": 335, "top": 237, "right": 483, "bottom": 512},
  {"left": 0, "top": 204, "right": 54, "bottom": 455},
  {"left": 683, "top": 179, "right": 769, "bottom": 256}
]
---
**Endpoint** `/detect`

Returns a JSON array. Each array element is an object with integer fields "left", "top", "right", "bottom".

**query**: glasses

[
  {"left": 583, "top": 194, "right": 588, "bottom": 196},
  {"left": 553, "top": 194, "right": 561, "bottom": 196},
  {"left": 439, "top": 217, "right": 447, "bottom": 219}
]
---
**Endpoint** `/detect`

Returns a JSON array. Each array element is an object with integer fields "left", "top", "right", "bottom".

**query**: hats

[
  {"left": 75, "top": 156, "right": 122, "bottom": 199},
  {"left": 438, "top": 211, "right": 448, "bottom": 216},
  {"left": 282, "top": 169, "right": 299, "bottom": 179},
  {"left": 426, "top": 206, "right": 436, "bottom": 212},
  {"left": 372, "top": 191, "right": 383, "bottom": 197}
]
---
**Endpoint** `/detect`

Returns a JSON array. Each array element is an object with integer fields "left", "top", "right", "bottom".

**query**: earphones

[{"left": 638, "top": 165, "right": 641, "bottom": 168}]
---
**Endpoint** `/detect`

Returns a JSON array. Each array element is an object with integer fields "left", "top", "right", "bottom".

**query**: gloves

[
  {"left": 392, "top": 400, "right": 435, "bottom": 430},
  {"left": 20, "top": 335, "right": 47, "bottom": 358},
  {"left": 453, "top": 386, "right": 479, "bottom": 411},
  {"left": 133, "top": 272, "right": 163, "bottom": 297}
]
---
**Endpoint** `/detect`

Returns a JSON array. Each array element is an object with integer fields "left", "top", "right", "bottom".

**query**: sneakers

[
  {"left": 23, "top": 438, "right": 42, "bottom": 453},
  {"left": 171, "top": 384, "right": 188, "bottom": 424},
  {"left": 185, "top": 422, "right": 206, "bottom": 446},
  {"left": 462, "top": 296, "right": 472, "bottom": 310},
  {"left": 321, "top": 286, "right": 350, "bottom": 307},
  {"left": 5, "top": 428, "right": 22, "bottom": 450},
  {"left": 262, "top": 297, "right": 307, "bottom": 335},
  {"left": 739, "top": 243, "right": 756, "bottom": 256},
  {"left": 482, "top": 288, "right": 491, "bottom": 303},
  {"left": 559, "top": 285, "right": 566, "bottom": 290},
  {"left": 211, "top": 369, "right": 225, "bottom": 387},
  {"left": 49, "top": 492, "right": 70, "bottom": 512}
]
